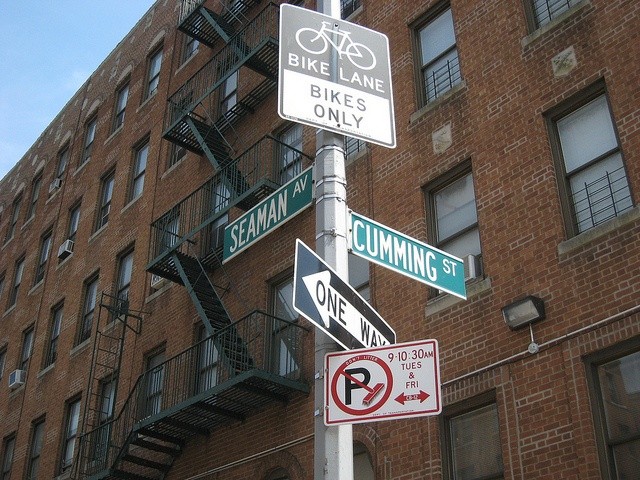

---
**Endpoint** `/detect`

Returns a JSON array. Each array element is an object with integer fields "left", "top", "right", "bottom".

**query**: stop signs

[{"left": 323, "top": 338, "right": 442, "bottom": 427}]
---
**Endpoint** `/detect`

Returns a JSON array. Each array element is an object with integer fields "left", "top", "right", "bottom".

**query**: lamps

[{"left": 500, "top": 295, "right": 546, "bottom": 356}]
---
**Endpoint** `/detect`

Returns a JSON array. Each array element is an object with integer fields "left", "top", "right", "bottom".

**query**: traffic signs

[
  {"left": 223, "top": 164, "right": 312, "bottom": 265},
  {"left": 292, "top": 237, "right": 397, "bottom": 353},
  {"left": 278, "top": 2, "right": 396, "bottom": 147},
  {"left": 345, "top": 205, "right": 468, "bottom": 301}
]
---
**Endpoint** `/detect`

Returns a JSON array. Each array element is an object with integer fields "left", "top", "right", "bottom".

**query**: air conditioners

[
  {"left": 8, "top": 369, "right": 26, "bottom": 392},
  {"left": 48, "top": 178, "right": 64, "bottom": 196},
  {"left": 57, "top": 239, "right": 75, "bottom": 261}
]
